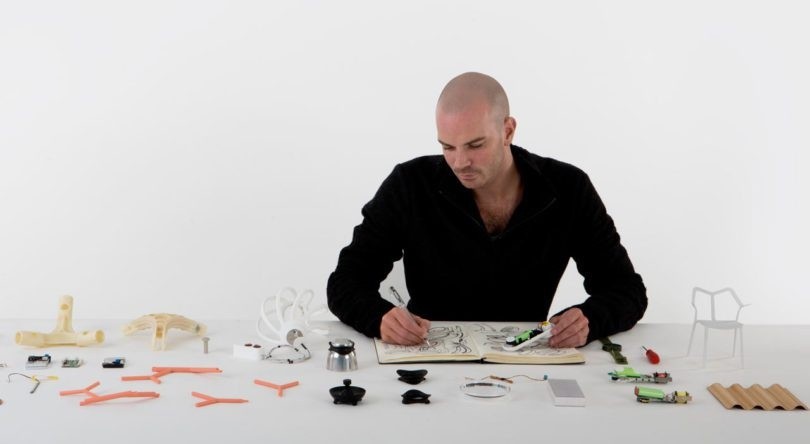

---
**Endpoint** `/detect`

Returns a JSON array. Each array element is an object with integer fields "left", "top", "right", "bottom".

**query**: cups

[{"left": 326, "top": 339, "right": 359, "bottom": 371}]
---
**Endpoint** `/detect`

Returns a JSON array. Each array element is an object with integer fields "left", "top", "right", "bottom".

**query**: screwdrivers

[{"left": 641, "top": 346, "right": 660, "bottom": 364}]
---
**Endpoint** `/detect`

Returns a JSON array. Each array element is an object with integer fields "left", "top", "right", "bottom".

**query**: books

[{"left": 373, "top": 321, "right": 585, "bottom": 365}]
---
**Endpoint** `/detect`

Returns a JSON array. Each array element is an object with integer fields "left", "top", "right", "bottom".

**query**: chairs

[{"left": 687, "top": 287, "right": 750, "bottom": 369}]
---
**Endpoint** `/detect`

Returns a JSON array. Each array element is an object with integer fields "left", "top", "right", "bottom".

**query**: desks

[{"left": 0, "top": 323, "right": 810, "bottom": 444}]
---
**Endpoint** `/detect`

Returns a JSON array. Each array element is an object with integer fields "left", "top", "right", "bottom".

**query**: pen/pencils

[{"left": 390, "top": 285, "right": 431, "bottom": 347}]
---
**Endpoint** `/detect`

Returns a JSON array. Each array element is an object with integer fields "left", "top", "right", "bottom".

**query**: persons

[{"left": 327, "top": 72, "right": 648, "bottom": 348}]
